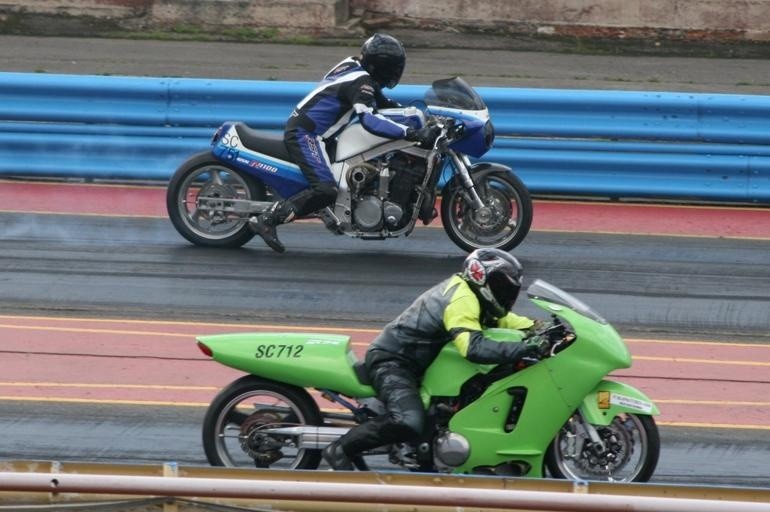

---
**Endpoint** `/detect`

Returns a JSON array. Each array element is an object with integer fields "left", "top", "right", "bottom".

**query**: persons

[
  {"left": 246, "top": 32, "right": 441, "bottom": 253},
  {"left": 320, "top": 247, "right": 554, "bottom": 471}
]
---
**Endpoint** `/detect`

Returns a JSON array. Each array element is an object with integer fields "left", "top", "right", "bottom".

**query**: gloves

[
  {"left": 405, "top": 126, "right": 440, "bottom": 150},
  {"left": 525, "top": 335, "right": 551, "bottom": 359}
]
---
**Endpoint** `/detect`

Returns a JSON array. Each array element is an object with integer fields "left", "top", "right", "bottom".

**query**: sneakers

[{"left": 323, "top": 441, "right": 355, "bottom": 471}]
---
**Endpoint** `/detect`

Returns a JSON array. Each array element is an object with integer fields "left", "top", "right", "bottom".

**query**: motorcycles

[
  {"left": 193, "top": 275, "right": 663, "bottom": 484},
  {"left": 164, "top": 73, "right": 534, "bottom": 255}
]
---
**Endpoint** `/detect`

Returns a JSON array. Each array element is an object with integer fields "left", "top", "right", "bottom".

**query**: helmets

[
  {"left": 361, "top": 32, "right": 406, "bottom": 89},
  {"left": 463, "top": 246, "right": 523, "bottom": 317}
]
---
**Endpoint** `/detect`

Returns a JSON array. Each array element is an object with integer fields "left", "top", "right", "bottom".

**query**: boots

[{"left": 249, "top": 199, "right": 296, "bottom": 253}]
